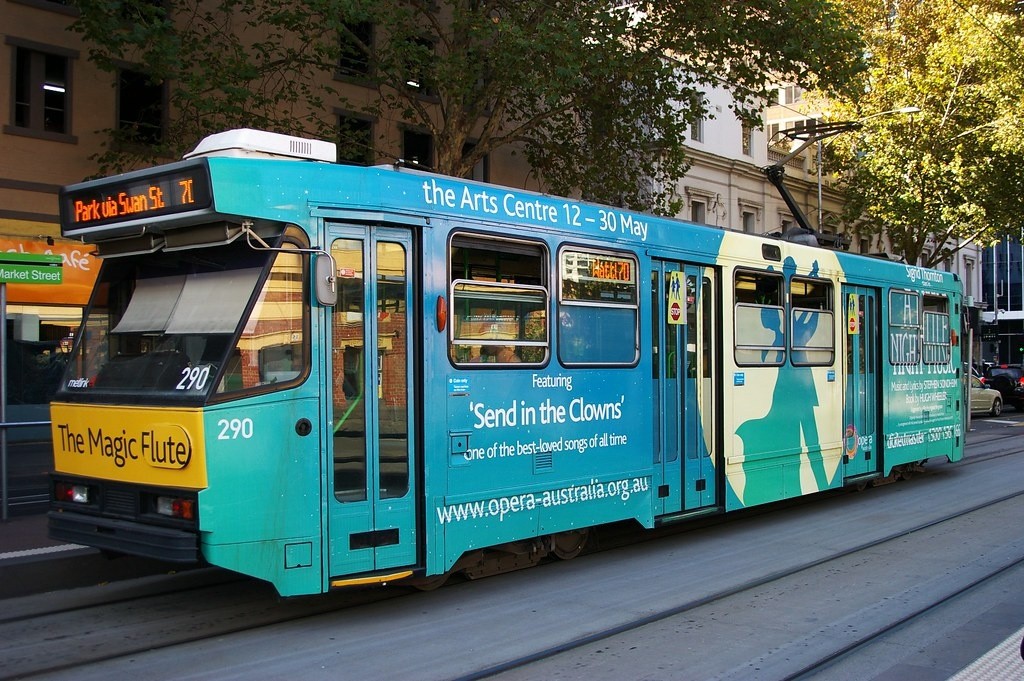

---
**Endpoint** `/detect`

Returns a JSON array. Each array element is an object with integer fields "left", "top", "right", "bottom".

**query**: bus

[{"left": 43, "top": 128, "right": 968, "bottom": 598}]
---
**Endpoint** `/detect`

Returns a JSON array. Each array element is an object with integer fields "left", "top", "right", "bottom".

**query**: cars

[
  {"left": 964, "top": 374, "right": 1002, "bottom": 416},
  {"left": 960, "top": 361, "right": 979, "bottom": 377}
]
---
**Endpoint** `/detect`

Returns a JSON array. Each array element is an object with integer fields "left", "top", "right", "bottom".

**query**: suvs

[{"left": 985, "top": 363, "right": 1024, "bottom": 412}]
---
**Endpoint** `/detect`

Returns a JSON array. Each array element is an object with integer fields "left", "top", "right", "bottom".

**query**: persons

[{"left": 483, "top": 345, "right": 521, "bottom": 362}]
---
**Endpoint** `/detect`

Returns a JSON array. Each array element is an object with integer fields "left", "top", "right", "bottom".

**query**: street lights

[{"left": 815, "top": 107, "right": 923, "bottom": 233}]
